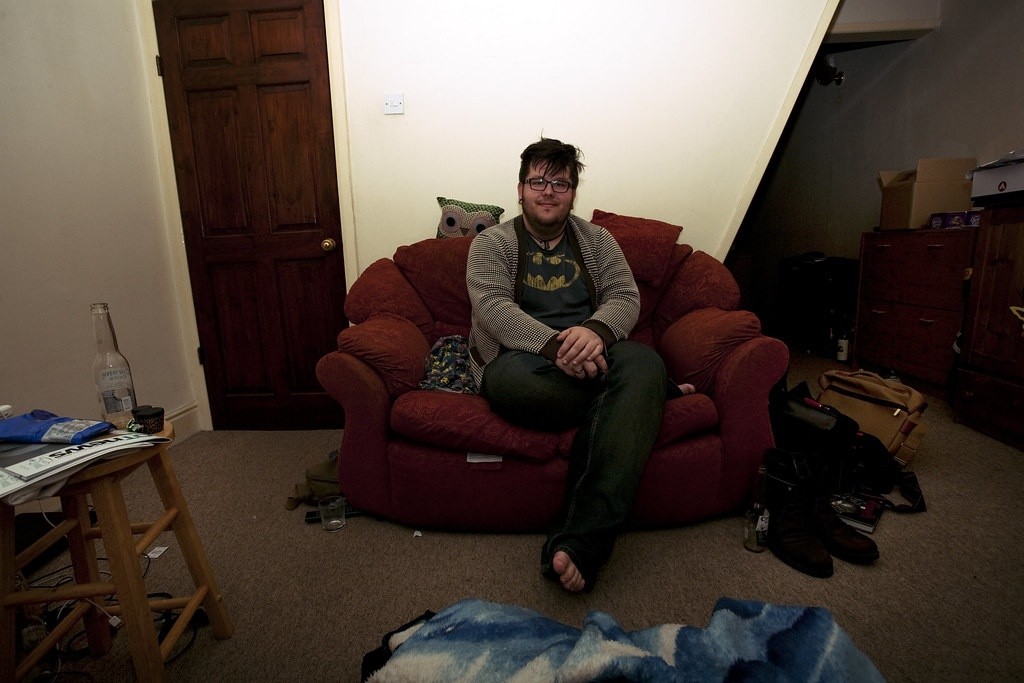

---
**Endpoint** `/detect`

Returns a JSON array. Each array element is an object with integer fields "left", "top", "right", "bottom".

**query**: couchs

[{"left": 315, "top": 208, "right": 793, "bottom": 535}]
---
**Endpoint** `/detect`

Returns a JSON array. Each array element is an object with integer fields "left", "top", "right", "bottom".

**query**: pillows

[
  {"left": 586, "top": 209, "right": 685, "bottom": 286},
  {"left": 434, "top": 196, "right": 505, "bottom": 238}
]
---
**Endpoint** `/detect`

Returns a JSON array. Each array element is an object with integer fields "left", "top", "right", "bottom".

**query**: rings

[{"left": 575, "top": 369, "right": 583, "bottom": 374}]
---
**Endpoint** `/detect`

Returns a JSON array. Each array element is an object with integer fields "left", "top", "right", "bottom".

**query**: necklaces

[{"left": 527, "top": 221, "right": 567, "bottom": 250}]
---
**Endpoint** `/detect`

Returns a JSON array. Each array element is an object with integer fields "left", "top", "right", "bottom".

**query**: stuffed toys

[{"left": 437, "top": 197, "right": 504, "bottom": 238}]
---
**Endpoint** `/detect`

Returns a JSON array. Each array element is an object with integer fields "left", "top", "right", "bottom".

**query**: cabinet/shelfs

[
  {"left": 850, "top": 230, "right": 977, "bottom": 389},
  {"left": 952, "top": 206, "right": 1024, "bottom": 440}
]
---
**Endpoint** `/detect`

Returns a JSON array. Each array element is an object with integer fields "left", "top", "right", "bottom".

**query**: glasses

[{"left": 525, "top": 177, "right": 572, "bottom": 192}]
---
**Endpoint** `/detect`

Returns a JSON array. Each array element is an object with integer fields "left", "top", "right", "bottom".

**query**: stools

[{"left": 0, "top": 420, "right": 235, "bottom": 683}]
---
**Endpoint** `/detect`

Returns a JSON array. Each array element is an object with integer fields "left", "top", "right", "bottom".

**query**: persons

[{"left": 466, "top": 139, "right": 696, "bottom": 597}]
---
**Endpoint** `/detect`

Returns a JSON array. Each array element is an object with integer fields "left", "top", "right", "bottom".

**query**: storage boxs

[{"left": 874, "top": 156, "right": 977, "bottom": 230}]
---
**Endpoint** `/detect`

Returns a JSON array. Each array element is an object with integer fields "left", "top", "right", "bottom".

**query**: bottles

[
  {"left": 742, "top": 501, "right": 769, "bottom": 552},
  {"left": 90, "top": 303, "right": 137, "bottom": 429},
  {"left": 22, "top": 616, "right": 52, "bottom": 675}
]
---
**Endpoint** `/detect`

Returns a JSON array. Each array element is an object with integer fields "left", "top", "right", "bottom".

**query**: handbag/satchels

[
  {"left": 286, "top": 448, "right": 340, "bottom": 510},
  {"left": 770, "top": 367, "right": 928, "bottom": 514}
]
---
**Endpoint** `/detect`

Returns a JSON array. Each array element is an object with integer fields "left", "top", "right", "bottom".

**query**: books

[
  {"left": 831, "top": 491, "right": 885, "bottom": 535},
  {"left": 0, "top": 430, "right": 172, "bottom": 498}
]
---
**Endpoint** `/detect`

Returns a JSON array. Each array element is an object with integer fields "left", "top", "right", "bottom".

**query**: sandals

[{"left": 541, "top": 531, "right": 600, "bottom": 595}]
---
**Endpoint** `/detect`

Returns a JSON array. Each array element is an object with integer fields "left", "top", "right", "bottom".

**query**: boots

[{"left": 757, "top": 470, "right": 879, "bottom": 578}]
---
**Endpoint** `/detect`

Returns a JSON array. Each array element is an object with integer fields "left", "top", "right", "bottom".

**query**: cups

[
  {"left": 318, "top": 496, "right": 346, "bottom": 532},
  {"left": 837, "top": 340, "right": 848, "bottom": 360}
]
